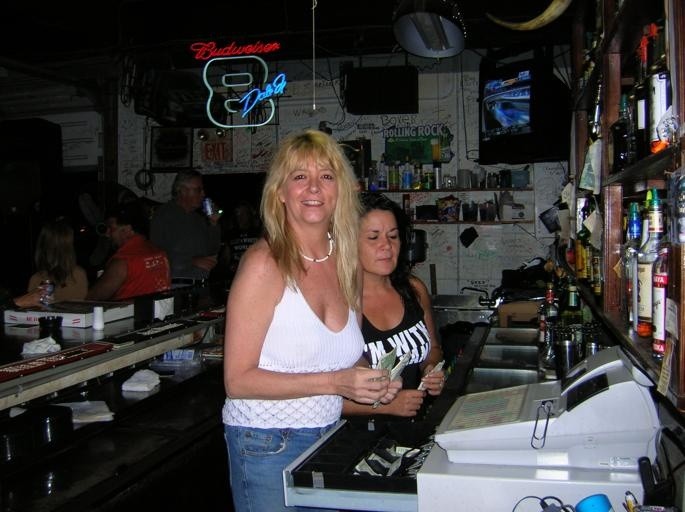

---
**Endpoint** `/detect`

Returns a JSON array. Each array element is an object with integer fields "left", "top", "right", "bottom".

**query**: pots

[{"left": 38, "top": 331, "right": 63, "bottom": 341}]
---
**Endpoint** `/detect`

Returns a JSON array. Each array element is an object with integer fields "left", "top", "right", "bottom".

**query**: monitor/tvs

[
  {"left": 344, "top": 65, "right": 419, "bottom": 115},
  {"left": 479, "top": 57, "right": 571, "bottom": 165}
]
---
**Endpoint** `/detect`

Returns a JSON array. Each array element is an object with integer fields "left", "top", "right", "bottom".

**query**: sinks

[
  {"left": 482, "top": 326, "right": 538, "bottom": 346},
  {"left": 461, "top": 369, "right": 538, "bottom": 392},
  {"left": 474, "top": 345, "right": 538, "bottom": 368}
]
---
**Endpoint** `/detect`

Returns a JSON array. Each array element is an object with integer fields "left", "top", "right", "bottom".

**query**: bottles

[
  {"left": 540, "top": 278, "right": 609, "bottom": 380},
  {"left": 368, "top": 156, "right": 499, "bottom": 190},
  {"left": 583, "top": 81, "right": 603, "bottom": 176},
  {"left": 91, "top": 305, "right": 106, "bottom": 330},
  {"left": 574, "top": 209, "right": 605, "bottom": 291},
  {"left": 92, "top": 331, "right": 104, "bottom": 343},
  {"left": 618, "top": 158, "right": 685, "bottom": 368},
  {"left": 40, "top": 280, "right": 54, "bottom": 304},
  {"left": 623, "top": 8, "right": 677, "bottom": 159},
  {"left": 403, "top": 194, "right": 411, "bottom": 219}
]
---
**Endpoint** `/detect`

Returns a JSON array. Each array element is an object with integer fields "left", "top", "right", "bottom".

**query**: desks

[{"left": 497, "top": 300, "right": 543, "bottom": 328}]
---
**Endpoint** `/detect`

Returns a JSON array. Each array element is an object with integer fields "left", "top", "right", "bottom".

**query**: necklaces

[{"left": 298, "top": 231, "right": 333, "bottom": 262}]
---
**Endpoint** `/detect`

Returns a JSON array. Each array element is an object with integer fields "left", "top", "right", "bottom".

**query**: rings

[
  {"left": 440, "top": 383, "right": 444, "bottom": 389},
  {"left": 441, "top": 377, "right": 445, "bottom": 384}
]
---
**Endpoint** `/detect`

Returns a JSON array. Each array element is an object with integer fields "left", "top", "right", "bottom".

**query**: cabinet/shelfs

[
  {"left": 360, "top": 162, "right": 536, "bottom": 241},
  {"left": 571, "top": 0, "right": 685, "bottom": 412}
]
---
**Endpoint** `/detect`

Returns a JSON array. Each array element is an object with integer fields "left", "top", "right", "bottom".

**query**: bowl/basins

[
  {"left": 435, "top": 199, "right": 461, "bottom": 223},
  {"left": 38, "top": 316, "right": 62, "bottom": 328}
]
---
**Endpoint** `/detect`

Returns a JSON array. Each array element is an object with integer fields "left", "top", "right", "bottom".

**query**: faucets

[{"left": 460, "top": 287, "right": 496, "bottom": 308}]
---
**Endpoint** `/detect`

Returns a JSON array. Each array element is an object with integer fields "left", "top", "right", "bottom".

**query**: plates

[{"left": 3, "top": 403, "right": 71, "bottom": 464}]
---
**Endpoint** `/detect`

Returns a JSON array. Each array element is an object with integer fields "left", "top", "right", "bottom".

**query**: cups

[
  {"left": 462, "top": 204, "right": 478, "bottom": 221},
  {"left": 497, "top": 169, "right": 530, "bottom": 190},
  {"left": 480, "top": 203, "right": 497, "bottom": 220},
  {"left": 430, "top": 139, "right": 441, "bottom": 160},
  {"left": 203, "top": 199, "right": 215, "bottom": 218}
]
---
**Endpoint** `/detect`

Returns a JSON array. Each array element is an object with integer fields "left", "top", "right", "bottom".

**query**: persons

[
  {"left": 224, "top": 204, "right": 261, "bottom": 259},
  {"left": 0, "top": 169, "right": 221, "bottom": 322},
  {"left": 220, "top": 128, "right": 403, "bottom": 512},
  {"left": 340, "top": 190, "right": 446, "bottom": 417}
]
